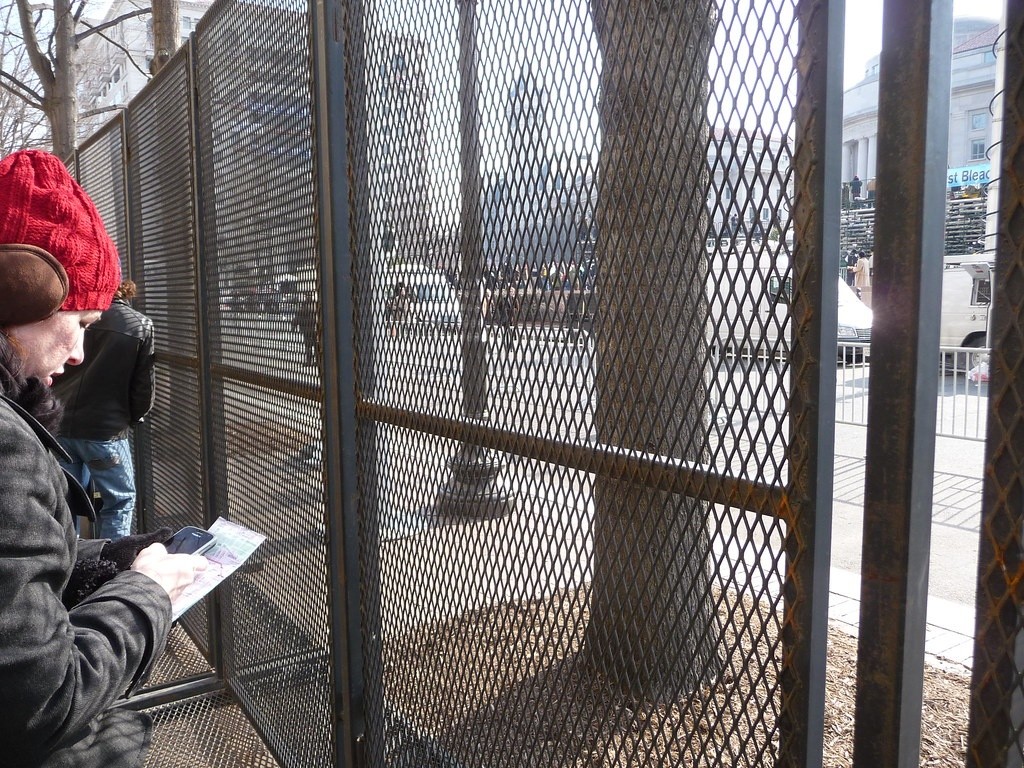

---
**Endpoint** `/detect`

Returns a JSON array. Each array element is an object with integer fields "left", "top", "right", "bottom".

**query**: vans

[
  {"left": 703, "top": 250, "right": 873, "bottom": 365},
  {"left": 386, "top": 261, "right": 462, "bottom": 333}
]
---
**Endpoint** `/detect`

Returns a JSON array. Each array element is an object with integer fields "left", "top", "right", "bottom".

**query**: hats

[{"left": 0, "top": 149, "right": 121, "bottom": 312}]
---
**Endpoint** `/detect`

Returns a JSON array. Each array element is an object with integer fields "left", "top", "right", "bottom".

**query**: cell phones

[{"left": 161, "top": 526, "right": 218, "bottom": 556}]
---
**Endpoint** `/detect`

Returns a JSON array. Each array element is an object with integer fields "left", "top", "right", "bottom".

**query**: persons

[
  {"left": 720, "top": 174, "right": 876, "bottom": 301},
  {"left": 0, "top": 149, "right": 591, "bottom": 768}
]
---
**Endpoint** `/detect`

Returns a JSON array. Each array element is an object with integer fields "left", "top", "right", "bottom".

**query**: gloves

[{"left": 99, "top": 527, "right": 173, "bottom": 572}]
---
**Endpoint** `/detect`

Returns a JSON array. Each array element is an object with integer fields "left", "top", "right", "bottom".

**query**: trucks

[{"left": 939, "top": 251, "right": 996, "bottom": 378}]
